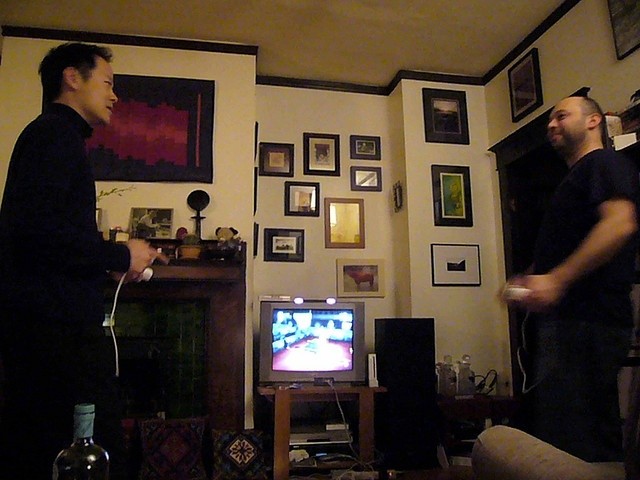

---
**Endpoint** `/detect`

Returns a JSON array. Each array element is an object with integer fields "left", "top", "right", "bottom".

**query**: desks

[{"left": 255, "top": 383, "right": 387, "bottom": 479}]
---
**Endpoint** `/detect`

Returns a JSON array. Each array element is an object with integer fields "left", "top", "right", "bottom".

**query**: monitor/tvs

[{"left": 260, "top": 301, "right": 365, "bottom": 383}]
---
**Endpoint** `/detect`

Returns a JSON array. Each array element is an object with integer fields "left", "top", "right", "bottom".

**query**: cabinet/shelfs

[{"left": 94, "top": 239, "right": 253, "bottom": 429}]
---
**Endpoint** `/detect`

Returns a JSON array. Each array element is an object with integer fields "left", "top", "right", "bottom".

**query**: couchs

[{"left": 473, "top": 420, "right": 636, "bottom": 474}]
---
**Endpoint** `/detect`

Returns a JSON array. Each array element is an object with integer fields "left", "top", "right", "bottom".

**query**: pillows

[
  {"left": 212, "top": 427, "right": 271, "bottom": 479},
  {"left": 137, "top": 416, "right": 209, "bottom": 479}
]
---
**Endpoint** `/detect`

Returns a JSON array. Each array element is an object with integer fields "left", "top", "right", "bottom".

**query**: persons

[
  {"left": 0, "top": 39, "right": 170, "bottom": 478},
  {"left": 497, "top": 93, "right": 639, "bottom": 458},
  {"left": 137, "top": 209, "right": 159, "bottom": 237}
]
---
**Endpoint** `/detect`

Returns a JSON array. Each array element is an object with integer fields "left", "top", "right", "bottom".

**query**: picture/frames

[
  {"left": 285, "top": 182, "right": 320, "bottom": 217},
  {"left": 324, "top": 197, "right": 365, "bottom": 248},
  {"left": 350, "top": 165, "right": 382, "bottom": 192},
  {"left": 422, "top": 87, "right": 470, "bottom": 144},
  {"left": 350, "top": 135, "right": 382, "bottom": 160},
  {"left": 507, "top": 47, "right": 544, "bottom": 123},
  {"left": 431, "top": 243, "right": 482, "bottom": 287},
  {"left": 391, "top": 180, "right": 402, "bottom": 213},
  {"left": 336, "top": 258, "right": 386, "bottom": 298},
  {"left": 259, "top": 143, "right": 295, "bottom": 177},
  {"left": 264, "top": 227, "right": 305, "bottom": 262},
  {"left": 95, "top": 208, "right": 101, "bottom": 231},
  {"left": 303, "top": 132, "right": 341, "bottom": 177},
  {"left": 128, "top": 207, "right": 175, "bottom": 240},
  {"left": 431, "top": 164, "right": 473, "bottom": 227}
]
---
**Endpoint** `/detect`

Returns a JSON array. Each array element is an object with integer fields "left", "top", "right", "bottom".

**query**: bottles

[{"left": 53, "top": 403, "right": 111, "bottom": 480}]
